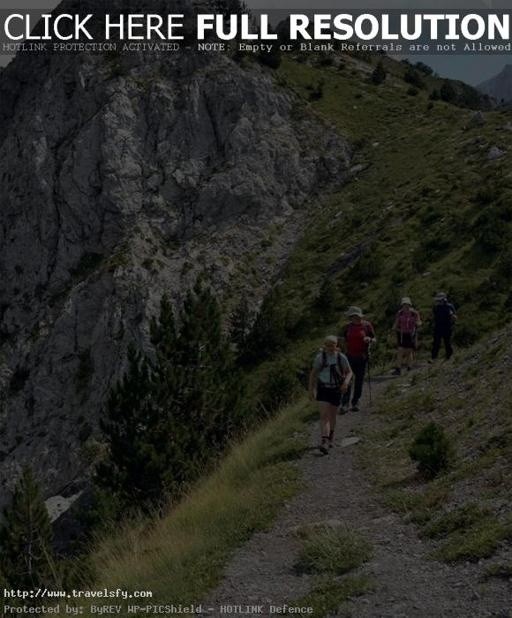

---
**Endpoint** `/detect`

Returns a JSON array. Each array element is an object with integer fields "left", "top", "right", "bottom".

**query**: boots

[
  {"left": 329, "top": 430, "right": 335, "bottom": 448},
  {"left": 320, "top": 436, "right": 329, "bottom": 453}
]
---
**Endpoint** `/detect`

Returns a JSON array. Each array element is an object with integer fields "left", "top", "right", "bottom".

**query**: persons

[
  {"left": 339, "top": 306, "right": 376, "bottom": 415},
  {"left": 308, "top": 335, "right": 353, "bottom": 455},
  {"left": 390, "top": 296, "right": 422, "bottom": 375},
  {"left": 428, "top": 292, "right": 457, "bottom": 363}
]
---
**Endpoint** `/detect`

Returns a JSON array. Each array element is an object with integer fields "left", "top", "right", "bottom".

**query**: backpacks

[{"left": 319, "top": 336, "right": 344, "bottom": 374}]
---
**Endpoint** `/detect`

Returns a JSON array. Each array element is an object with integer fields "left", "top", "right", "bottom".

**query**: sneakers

[
  {"left": 340, "top": 405, "right": 349, "bottom": 414},
  {"left": 392, "top": 367, "right": 400, "bottom": 374},
  {"left": 352, "top": 404, "right": 361, "bottom": 410}
]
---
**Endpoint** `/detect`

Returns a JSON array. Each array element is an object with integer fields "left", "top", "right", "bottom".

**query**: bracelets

[{"left": 370, "top": 338, "right": 372, "bottom": 343}]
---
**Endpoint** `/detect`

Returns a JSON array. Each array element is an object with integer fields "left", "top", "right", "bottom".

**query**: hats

[
  {"left": 323, "top": 334, "right": 337, "bottom": 343},
  {"left": 401, "top": 296, "right": 412, "bottom": 305},
  {"left": 434, "top": 292, "right": 447, "bottom": 301},
  {"left": 344, "top": 305, "right": 366, "bottom": 317}
]
